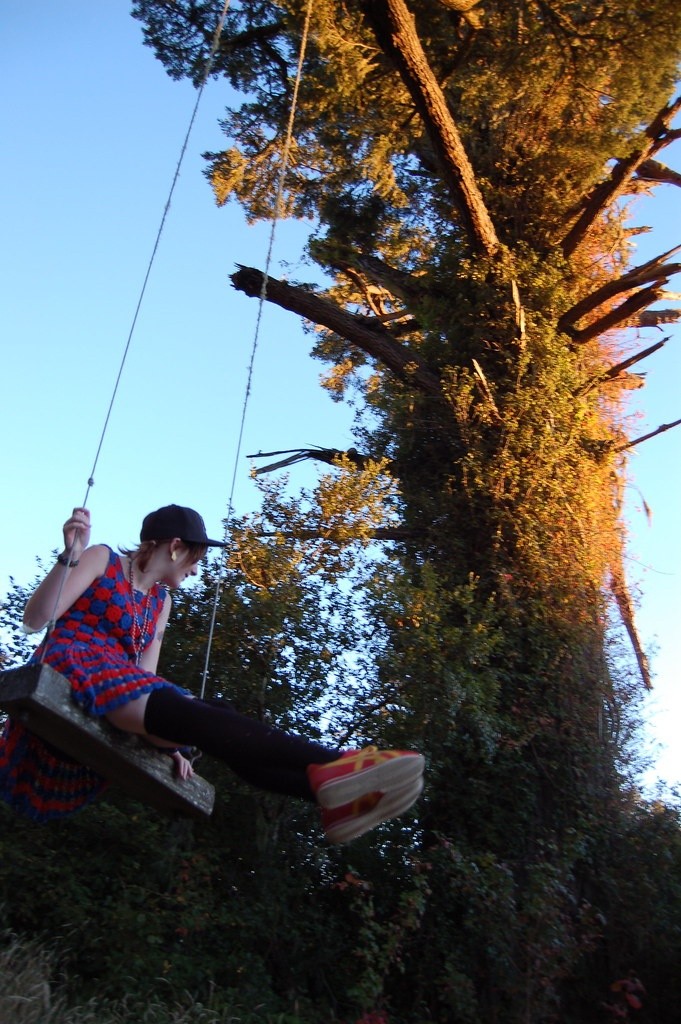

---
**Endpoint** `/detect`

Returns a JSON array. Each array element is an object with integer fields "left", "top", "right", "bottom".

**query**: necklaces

[{"left": 126, "top": 557, "right": 152, "bottom": 667}]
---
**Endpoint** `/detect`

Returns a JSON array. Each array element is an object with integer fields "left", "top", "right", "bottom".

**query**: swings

[{"left": 0, "top": 1, "right": 315, "bottom": 821}]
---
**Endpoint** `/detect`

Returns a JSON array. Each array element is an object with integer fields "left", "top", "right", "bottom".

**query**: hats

[{"left": 141, "top": 505, "right": 230, "bottom": 548}]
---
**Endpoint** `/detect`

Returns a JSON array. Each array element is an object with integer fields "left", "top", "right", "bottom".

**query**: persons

[{"left": 0, "top": 502, "right": 424, "bottom": 844}]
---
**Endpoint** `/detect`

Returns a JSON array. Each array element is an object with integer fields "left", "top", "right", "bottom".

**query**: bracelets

[{"left": 55, "top": 554, "right": 79, "bottom": 568}]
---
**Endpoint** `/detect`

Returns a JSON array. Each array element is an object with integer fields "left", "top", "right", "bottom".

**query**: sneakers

[
  {"left": 320, "top": 775, "right": 424, "bottom": 843},
  {"left": 308, "top": 745, "right": 425, "bottom": 810}
]
